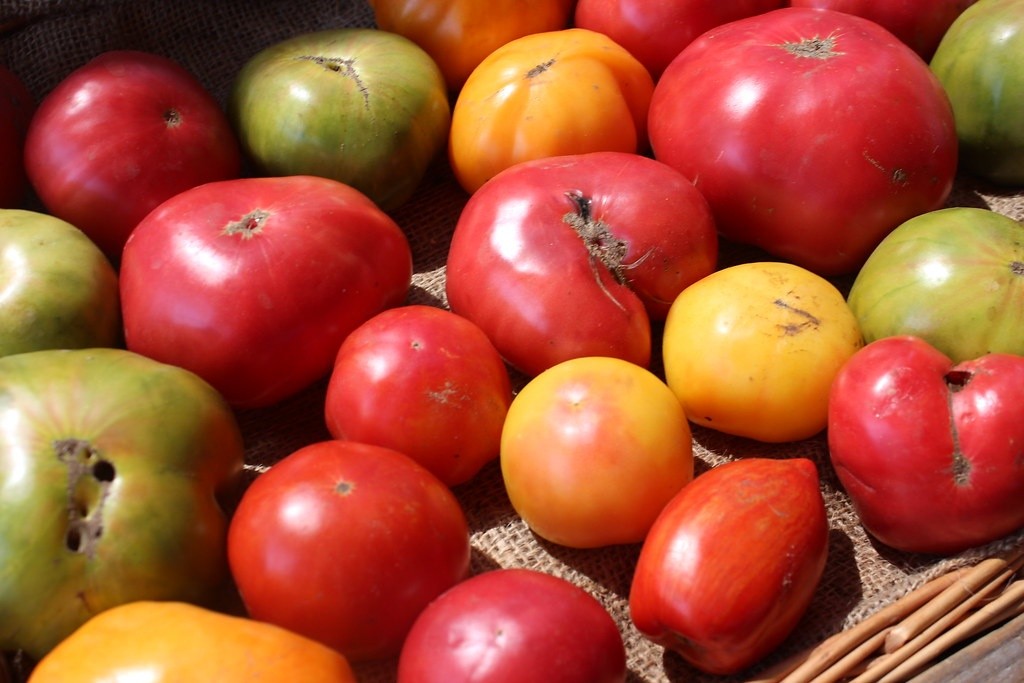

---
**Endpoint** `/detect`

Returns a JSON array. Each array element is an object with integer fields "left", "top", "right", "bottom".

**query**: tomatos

[{"left": 0, "top": 0, "right": 1024, "bottom": 683}]
